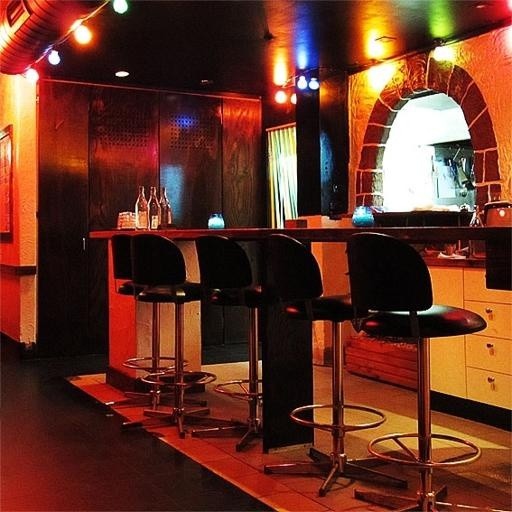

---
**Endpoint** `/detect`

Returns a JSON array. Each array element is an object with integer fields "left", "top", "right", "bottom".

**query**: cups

[
  {"left": 117, "top": 211, "right": 135, "bottom": 230},
  {"left": 445, "top": 243, "right": 454, "bottom": 255}
]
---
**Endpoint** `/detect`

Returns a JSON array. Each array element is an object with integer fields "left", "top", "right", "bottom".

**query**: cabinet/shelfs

[{"left": 424, "top": 265, "right": 512, "bottom": 413}]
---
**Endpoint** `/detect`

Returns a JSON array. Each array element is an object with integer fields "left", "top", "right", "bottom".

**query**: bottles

[
  {"left": 134, "top": 186, "right": 149, "bottom": 231},
  {"left": 468, "top": 205, "right": 486, "bottom": 259},
  {"left": 147, "top": 187, "right": 163, "bottom": 230},
  {"left": 158, "top": 186, "right": 173, "bottom": 228}
]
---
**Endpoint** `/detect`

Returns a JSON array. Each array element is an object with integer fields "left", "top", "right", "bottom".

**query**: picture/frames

[{"left": 1, "top": 123, "right": 16, "bottom": 244}]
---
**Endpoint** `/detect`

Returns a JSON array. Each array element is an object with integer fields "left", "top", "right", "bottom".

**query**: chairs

[
  {"left": 121, "top": 235, "right": 239, "bottom": 438},
  {"left": 190, "top": 238, "right": 265, "bottom": 451},
  {"left": 104, "top": 234, "right": 208, "bottom": 412},
  {"left": 342, "top": 229, "right": 511, "bottom": 512},
  {"left": 253, "top": 230, "right": 411, "bottom": 498}
]
